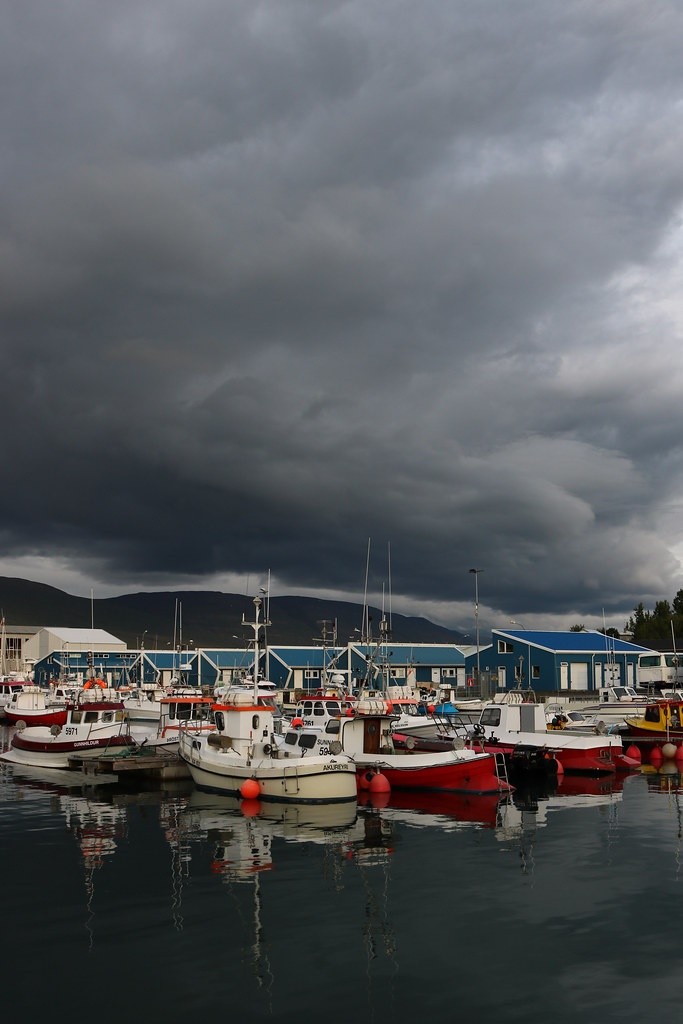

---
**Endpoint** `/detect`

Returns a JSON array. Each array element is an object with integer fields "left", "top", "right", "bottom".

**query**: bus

[{"left": 637, "top": 653, "right": 683, "bottom": 689}]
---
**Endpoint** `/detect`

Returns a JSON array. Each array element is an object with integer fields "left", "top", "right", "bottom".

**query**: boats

[{"left": 1, "top": 540, "right": 683, "bottom": 829}]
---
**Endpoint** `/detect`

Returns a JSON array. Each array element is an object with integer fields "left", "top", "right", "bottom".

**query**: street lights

[{"left": 469, "top": 567, "right": 484, "bottom": 698}]
